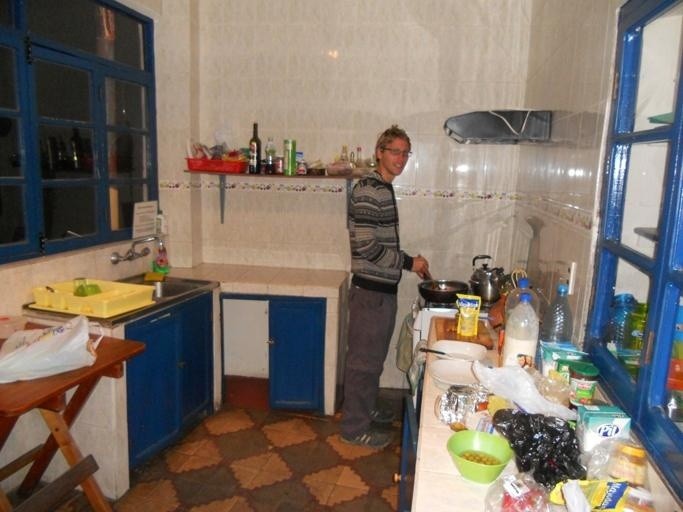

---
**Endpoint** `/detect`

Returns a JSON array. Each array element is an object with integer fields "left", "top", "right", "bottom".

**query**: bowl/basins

[
  {"left": 429, "top": 360, "right": 478, "bottom": 390},
  {"left": 447, "top": 430, "right": 514, "bottom": 483}
]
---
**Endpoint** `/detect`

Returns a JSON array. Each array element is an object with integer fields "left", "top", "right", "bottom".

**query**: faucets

[{"left": 111, "top": 235, "right": 163, "bottom": 264}]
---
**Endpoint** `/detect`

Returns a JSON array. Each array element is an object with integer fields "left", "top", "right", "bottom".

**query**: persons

[{"left": 339, "top": 127, "right": 429, "bottom": 448}]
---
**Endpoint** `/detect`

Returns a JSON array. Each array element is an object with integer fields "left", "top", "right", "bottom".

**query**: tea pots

[
  {"left": 470, "top": 255, "right": 505, "bottom": 303},
  {"left": 435, "top": 384, "right": 488, "bottom": 423}
]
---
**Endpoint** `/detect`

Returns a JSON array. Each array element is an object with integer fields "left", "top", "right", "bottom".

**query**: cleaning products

[
  {"left": 153, "top": 239, "right": 169, "bottom": 275},
  {"left": 454, "top": 291, "right": 481, "bottom": 338},
  {"left": 151, "top": 236, "right": 170, "bottom": 273},
  {"left": 549, "top": 480, "right": 630, "bottom": 511}
]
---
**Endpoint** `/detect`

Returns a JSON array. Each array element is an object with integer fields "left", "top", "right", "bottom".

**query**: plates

[
  {"left": 432, "top": 340, "right": 487, "bottom": 362},
  {"left": 32, "top": 278, "right": 156, "bottom": 318},
  {"left": 28, "top": 301, "right": 157, "bottom": 318}
]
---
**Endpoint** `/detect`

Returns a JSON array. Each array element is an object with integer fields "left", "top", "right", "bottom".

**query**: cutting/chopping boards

[{"left": 435, "top": 317, "right": 493, "bottom": 350}]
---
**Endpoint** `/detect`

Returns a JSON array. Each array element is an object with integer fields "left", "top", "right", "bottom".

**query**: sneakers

[
  {"left": 339, "top": 429, "right": 393, "bottom": 448},
  {"left": 368, "top": 407, "right": 397, "bottom": 424}
]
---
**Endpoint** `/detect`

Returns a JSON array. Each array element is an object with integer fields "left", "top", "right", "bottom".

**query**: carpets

[{"left": 111, "top": 404, "right": 399, "bottom": 512}]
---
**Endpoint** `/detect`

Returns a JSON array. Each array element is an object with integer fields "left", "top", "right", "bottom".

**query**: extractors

[{"left": 444, "top": 110, "right": 552, "bottom": 144}]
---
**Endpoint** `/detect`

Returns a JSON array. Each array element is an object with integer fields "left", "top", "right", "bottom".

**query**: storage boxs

[
  {"left": 393, "top": 290, "right": 683, "bottom": 512},
  {"left": 20, "top": 263, "right": 349, "bottom": 500}
]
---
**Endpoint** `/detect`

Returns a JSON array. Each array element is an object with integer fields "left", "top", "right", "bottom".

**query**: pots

[{"left": 417, "top": 272, "right": 469, "bottom": 304}]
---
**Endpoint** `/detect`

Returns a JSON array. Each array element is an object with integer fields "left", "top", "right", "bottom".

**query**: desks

[{"left": 0, "top": 318, "right": 146, "bottom": 512}]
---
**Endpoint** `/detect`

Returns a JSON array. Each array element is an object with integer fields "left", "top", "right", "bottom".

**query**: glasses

[{"left": 384, "top": 147, "right": 413, "bottom": 158}]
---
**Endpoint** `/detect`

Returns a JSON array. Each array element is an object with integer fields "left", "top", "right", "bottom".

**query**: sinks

[{"left": 117, "top": 270, "right": 219, "bottom": 300}]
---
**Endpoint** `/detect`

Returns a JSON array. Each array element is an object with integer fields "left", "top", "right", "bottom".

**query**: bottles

[
  {"left": 504, "top": 279, "right": 539, "bottom": 327},
  {"left": 276, "top": 154, "right": 285, "bottom": 176},
  {"left": 567, "top": 365, "right": 600, "bottom": 411},
  {"left": 535, "top": 285, "right": 573, "bottom": 371},
  {"left": 350, "top": 152, "right": 356, "bottom": 170},
  {"left": 608, "top": 294, "right": 631, "bottom": 353},
  {"left": 355, "top": 147, "right": 362, "bottom": 167},
  {"left": 500, "top": 292, "right": 538, "bottom": 371},
  {"left": 619, "top": 302, "right": 648, "bottom": 375},
  {"left": 250, "top": 123, "right": 261, "bottom": 176},
  {"left": 297, "top": 159, "right": 307, "bottom": 175},
  {"left": 265, "top": 138, "right": 276, "bottom": 176},
  {"left": 284, "top": 140, "right": 296, "bottom": 175},
  {"left": 340, "top": 144, "right": 348, "bottom": 161},
  {"left": 608, "top": 442, "right": 646, "bottom": 491}
]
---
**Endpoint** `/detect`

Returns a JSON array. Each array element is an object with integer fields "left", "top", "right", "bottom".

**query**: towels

[
  {"left": 407, "top": 338, "right": 428, "bottom": 396},
  {"left": 395, "top": 313, "right": 416, "bottom": 373}
]
